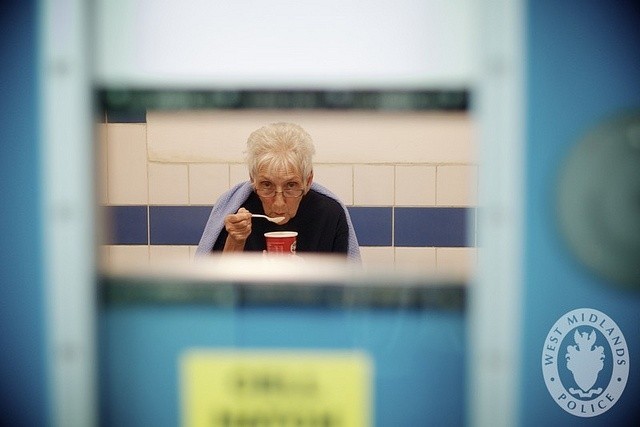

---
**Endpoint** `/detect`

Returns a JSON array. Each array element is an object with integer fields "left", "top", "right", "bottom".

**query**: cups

[{"left": 264, "top": 231, "right": 298, "bottom": 256}]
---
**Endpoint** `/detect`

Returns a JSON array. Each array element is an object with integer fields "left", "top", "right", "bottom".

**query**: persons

[{"left": 190, "top": 121, "right": 367, "bottom": 286}]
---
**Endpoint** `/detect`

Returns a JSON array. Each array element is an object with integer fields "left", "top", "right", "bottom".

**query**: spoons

[{"left": 252, "top": 214, "right": 286, "bottom": 223}]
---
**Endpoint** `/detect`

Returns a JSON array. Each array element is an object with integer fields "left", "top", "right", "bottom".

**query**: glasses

[{"left": 254, "top": 180, "right": 306, "bottom": 198}]
